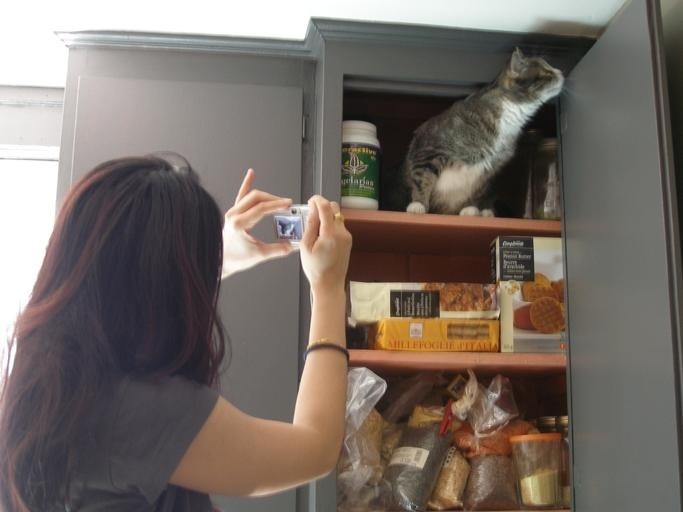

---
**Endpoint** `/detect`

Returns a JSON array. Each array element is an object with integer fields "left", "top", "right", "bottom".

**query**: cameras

[{"left": 272, "top": 204, "right": 309, "bottom": 249}]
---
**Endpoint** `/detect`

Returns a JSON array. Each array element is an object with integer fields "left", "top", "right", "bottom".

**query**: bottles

[
  {"left": 537, "top": 415, "right": 573, "bottom": 486},
  {"left": 532, "top": 137, "right": 563, "bottom": 220},
  {"left": 339, "top": 117, "right": 380, "bottom": 208}
]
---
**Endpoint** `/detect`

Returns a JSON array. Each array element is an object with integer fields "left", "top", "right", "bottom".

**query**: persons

[{"left": 0, "top": 149, "right": 351, "bottom": 512}]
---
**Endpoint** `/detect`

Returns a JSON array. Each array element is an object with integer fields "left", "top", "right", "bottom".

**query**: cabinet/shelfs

[{"left": 336, "top": 1, "right": 683, "bottom": 512}]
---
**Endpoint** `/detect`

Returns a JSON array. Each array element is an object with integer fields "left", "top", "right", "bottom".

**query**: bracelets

[{"left": 303, "top": 338, "right": 349, "bottom": 363}]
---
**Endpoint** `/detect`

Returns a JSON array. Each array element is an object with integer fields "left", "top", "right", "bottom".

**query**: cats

[{"left": 388, "top": 46, "right": 575, "bottom": 218}]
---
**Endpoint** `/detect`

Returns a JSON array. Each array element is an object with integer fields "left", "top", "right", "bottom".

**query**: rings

[{"left": 333, "top": 211, "right": 345, "bottom": 221}]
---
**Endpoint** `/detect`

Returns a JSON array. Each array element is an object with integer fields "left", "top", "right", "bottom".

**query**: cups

[{"left": 508, "top": 432, "right": 570, "bottom": 509}]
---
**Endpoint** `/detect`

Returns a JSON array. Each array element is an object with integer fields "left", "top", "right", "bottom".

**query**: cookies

[{"left": 523, "top": 273, "right": 564, "bottom": 333}]
